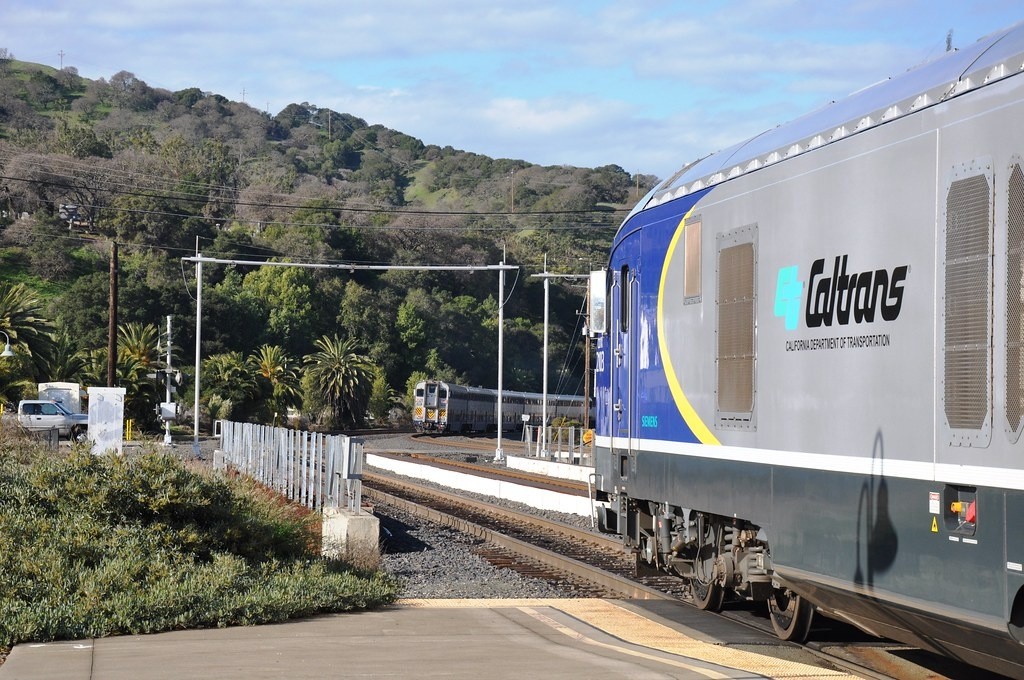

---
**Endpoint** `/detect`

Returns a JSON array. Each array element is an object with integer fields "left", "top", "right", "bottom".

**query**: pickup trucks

[{"left": 2, "top": 400, "right": 88, "bottom": 440}]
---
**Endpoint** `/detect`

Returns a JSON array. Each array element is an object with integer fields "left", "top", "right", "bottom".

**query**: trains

[
  {"left": 583, "top": 24, "right": 1024, "bottom": 680},
  {"left": 412, "top": 381, "right": 596, "bottom": 434}
]
